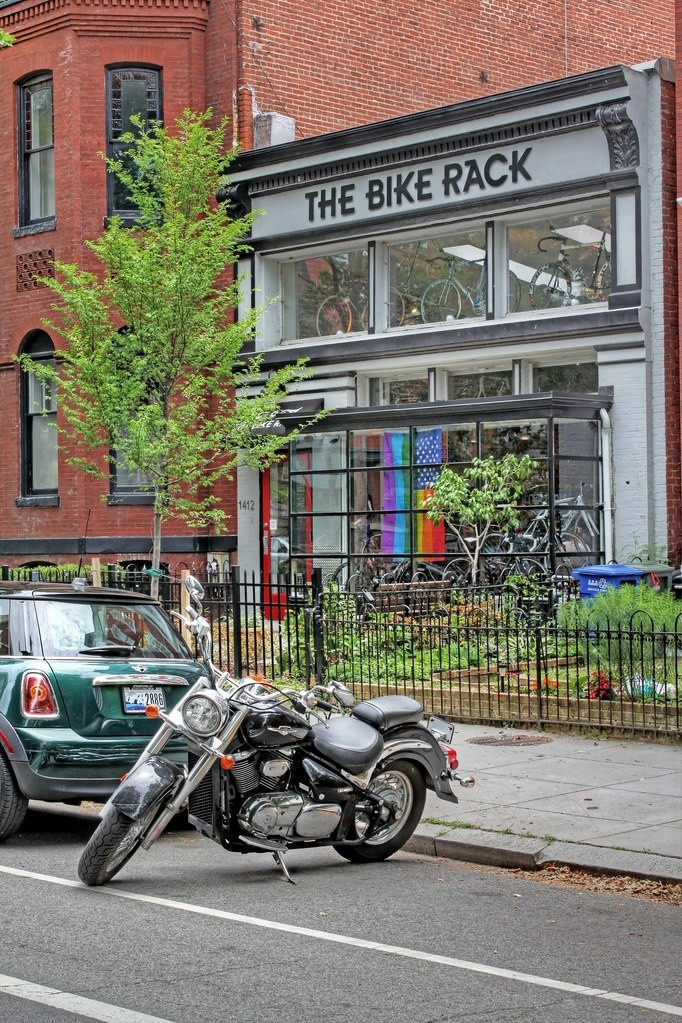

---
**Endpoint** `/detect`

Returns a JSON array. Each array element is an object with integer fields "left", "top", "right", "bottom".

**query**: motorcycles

[{"left": 77, "top": 574, "right": 474, "bottom": 887}]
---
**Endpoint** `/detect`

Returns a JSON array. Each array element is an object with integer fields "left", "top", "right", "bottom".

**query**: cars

[{"left": 0, "top": 579, "right": 213, "bottom": 837}]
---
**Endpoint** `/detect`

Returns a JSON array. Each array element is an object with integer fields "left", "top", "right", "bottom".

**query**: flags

[{"left": 378, "top": 426, "right": 445, "bottom": 563}]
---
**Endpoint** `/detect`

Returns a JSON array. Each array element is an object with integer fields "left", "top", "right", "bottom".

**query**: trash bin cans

[
  {"left": 571, "top": 564, "right": 643, "bottom": 645},
  {"left": 625, "top": 561, "right": 676, "bottom": 600}
]
